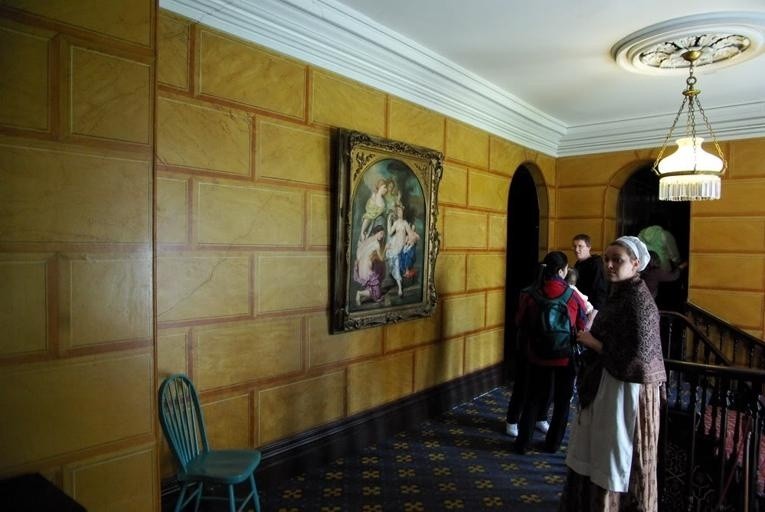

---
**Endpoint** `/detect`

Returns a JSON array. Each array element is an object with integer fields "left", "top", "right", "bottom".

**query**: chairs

[{"left": 157, "top": 374, "right": 262, "bottom": 511}]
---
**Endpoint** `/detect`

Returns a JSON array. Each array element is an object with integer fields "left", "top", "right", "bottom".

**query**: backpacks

[{"left": 523, "top": 286, "right": 575, "bottom": 360}]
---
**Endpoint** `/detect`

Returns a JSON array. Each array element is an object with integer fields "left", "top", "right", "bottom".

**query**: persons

[
  {"left": 639, "top": 250, "right": 688, "bottom": 298},
  {"left": 353, "top": 168, "right": 420, "bottom": 308},
  {"left": 570, "top": 234, "right": 604, "bottom": 308},
  {"left": 559, "top": 235, "right": 671, "bottom": 512},
  {"left": 506, "top": 251, "right": 598, "bottom": 454}
]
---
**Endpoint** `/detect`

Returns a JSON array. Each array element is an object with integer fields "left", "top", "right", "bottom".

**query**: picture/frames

[{"left": 329, "top": 128, "right": 444, "bottom": 334}]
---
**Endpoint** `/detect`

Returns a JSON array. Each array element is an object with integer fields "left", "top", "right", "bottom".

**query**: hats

[{"left": 613, "top": 235, "right": 651, "bottom": 272}]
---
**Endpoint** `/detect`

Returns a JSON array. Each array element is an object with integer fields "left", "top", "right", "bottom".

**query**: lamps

[{"left": 609, "top": 10, "right": 764, "bottom": 202}]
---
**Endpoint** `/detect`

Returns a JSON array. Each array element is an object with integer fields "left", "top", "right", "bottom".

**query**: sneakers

[{"left": 506, "top": 421, "right": 556, "bottom": 453}]
